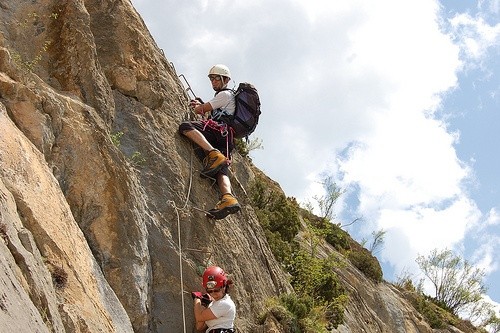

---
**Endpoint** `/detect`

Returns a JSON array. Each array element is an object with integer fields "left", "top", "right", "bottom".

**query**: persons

[
  {"left": 178, "top": 65, "right": 241, "bottom": 221},
  {"left": 192, "top": 266, "right": 236, "bottom": 333}
]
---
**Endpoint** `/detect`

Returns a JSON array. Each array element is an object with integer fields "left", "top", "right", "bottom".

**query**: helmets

[
  {"left": 203, "top": 266, "right": 227, "bottom": 288},
  {"left": 209, "top": 64, "right": 231, "bottom": 80}
]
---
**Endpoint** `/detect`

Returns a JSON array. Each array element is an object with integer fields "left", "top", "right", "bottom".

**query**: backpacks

[{"left": 214, "top": 82, "right": 261, "bottom": 139}]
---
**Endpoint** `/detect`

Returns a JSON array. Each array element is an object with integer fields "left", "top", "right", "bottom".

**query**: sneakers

[
  {"left": 207, "top": 193, "right": 241, "bottom": 220},
  {"left": 200, "top": 149, "right": 229, "bottom": 178}
]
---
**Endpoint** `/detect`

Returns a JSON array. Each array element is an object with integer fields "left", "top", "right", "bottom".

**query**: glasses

[
  {"left": 207, "top": 286, "right": 224, "bottom": 293},
  {"left": 210, "top": 76, "right": 227, "bottom": 81}
]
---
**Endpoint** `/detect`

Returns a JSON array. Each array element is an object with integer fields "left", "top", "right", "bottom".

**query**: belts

[{"left": 209, "top": 328, "right": 233, "bottom": 333}]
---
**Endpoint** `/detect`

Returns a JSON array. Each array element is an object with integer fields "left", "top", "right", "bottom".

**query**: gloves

[
  {"left": 188, "top": 97, "right": 204, "bottom": 109},
  {"left": 202, "top": 293, "right": 213, "bottom": 304},
  {"left": 192, "top": 292, "right": 202, "bottom": 302}
]
